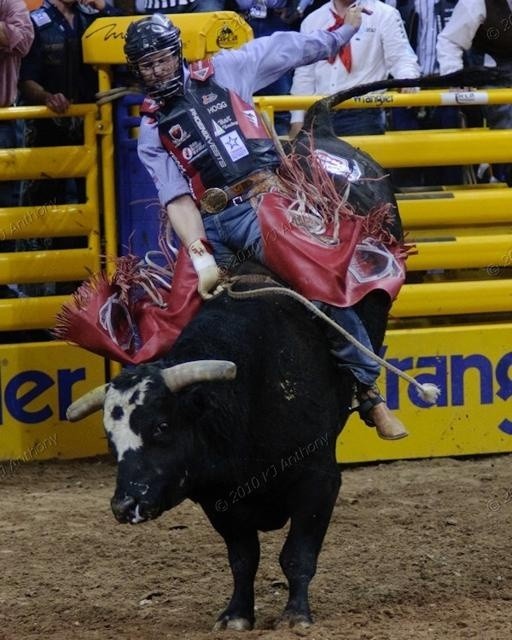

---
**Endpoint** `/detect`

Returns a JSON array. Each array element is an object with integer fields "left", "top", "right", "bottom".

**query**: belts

[{"left": 197, "top": 170, "right": 267, "bottom": 220}]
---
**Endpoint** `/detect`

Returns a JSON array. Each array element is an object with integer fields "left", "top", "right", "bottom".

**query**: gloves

[{"left": 193, "top": 255, "right": 226, "bottom": 302}]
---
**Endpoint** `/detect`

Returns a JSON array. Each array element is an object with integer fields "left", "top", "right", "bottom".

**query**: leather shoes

[{"left": 355, "top": 387, "right": 407, "bottom": 441}]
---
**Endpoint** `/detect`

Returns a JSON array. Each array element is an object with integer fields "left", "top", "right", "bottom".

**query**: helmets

[{"left": 123, "top": 14, "right": 185, "bottom": 103}]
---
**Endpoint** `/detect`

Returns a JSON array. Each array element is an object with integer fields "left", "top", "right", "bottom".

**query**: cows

[{"left": 61, "top": 63, "right": 512, "bottom": 634}]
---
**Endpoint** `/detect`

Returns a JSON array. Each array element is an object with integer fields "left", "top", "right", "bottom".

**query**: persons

[
  {"left": 0, "top": 1, "right": 512, "bottom": 298},
  {"left": 48, "top": 6, "right": 422, "bottom": 442}
]
